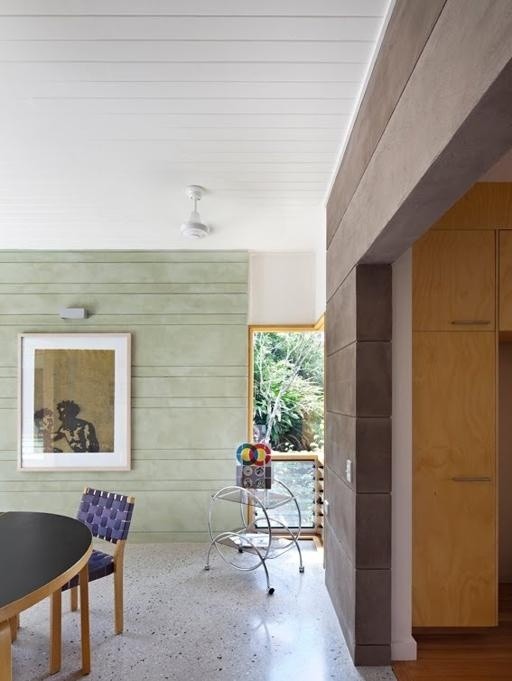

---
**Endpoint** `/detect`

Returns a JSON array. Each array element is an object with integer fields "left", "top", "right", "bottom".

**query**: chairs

[{"left": 46, "top": 485, "right": 136, "bottom": 676}]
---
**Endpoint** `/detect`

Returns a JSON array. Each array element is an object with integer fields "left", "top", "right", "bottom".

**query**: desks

[{"left": 0, "top": 510, "right": 94, "bottom": 680}]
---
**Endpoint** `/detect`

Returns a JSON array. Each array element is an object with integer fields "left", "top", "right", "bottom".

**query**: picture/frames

[{"left": 17, "top": 332, "right": 132, "bottom": 473}]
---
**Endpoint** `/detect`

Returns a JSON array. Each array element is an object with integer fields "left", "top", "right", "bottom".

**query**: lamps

[{"left": 179, "top": 186, "right": 210, "bottom": 241}]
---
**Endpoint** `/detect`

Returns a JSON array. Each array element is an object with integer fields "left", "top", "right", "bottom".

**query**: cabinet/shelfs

[
  {"left": 203, "top": 477, "right": 305, "bottom": 595},
  {"left": 411, "top": 182, "right": 499, "bottom": 636}
]
